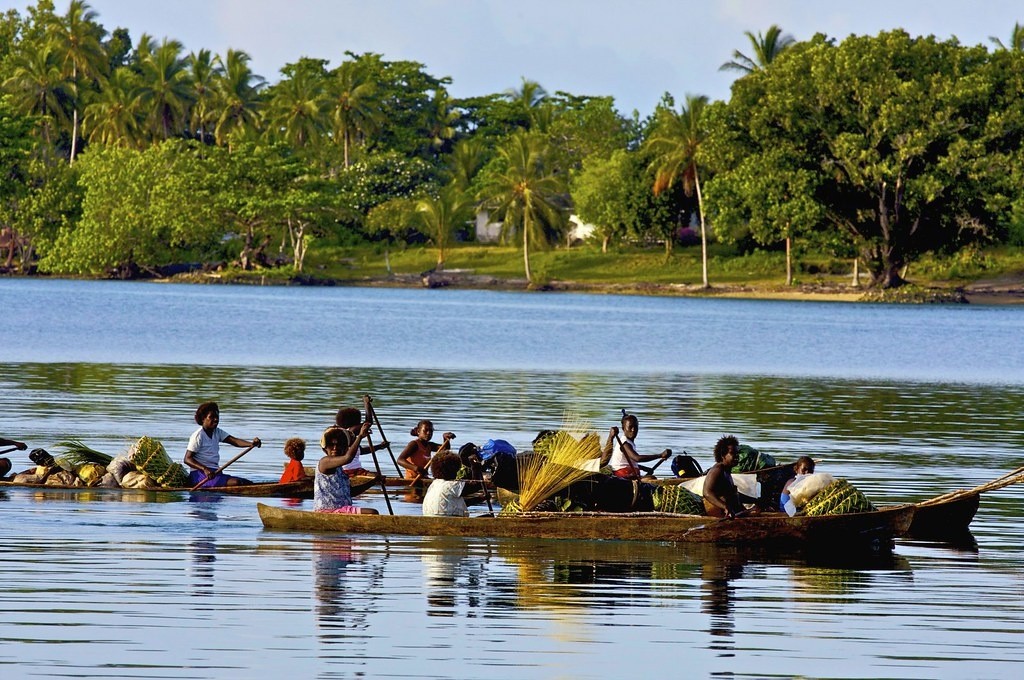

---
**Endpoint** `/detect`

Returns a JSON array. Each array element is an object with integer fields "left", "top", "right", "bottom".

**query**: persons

[
  {"left": 0, "top": 438, "right": 28, "bottom": 481},
  {"left": 336, "top": 394, "right": 390, "bottom": 478},
  {"left": 422, "top": 451, "right": 493, "bottom": 517},
  {"left": 608, "top": 408, "right": 672, "bottom": 478},
  {"left": 397, "top": 420, "right": 456, "bottom": 479},
  {"left": 456, "top": 443, "right": 486, "bottom": 480},
  {"left": 278, "top": 438, "right": 313, "bottom": 485},
  {"left": 183, "top": 402, "right": 263, "bottom": 488},
  {"left": 701, "top": 437, "right": 765, "bottom": 517},
  {"left": 575, "top": 426, "right": 619, "bottom": 474},
  {"left": 780, "top": 455, "right": 816, "bottom": 513},
  {"left": 313, "top": 421, "right": 387, "bottom": 515}
]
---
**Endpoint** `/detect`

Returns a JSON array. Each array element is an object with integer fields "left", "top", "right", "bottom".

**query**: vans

[{"left": 476, "top": 201, "right": 600, "bottom": 248}]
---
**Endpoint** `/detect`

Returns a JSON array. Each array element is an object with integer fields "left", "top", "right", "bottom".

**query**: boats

[
  {"left": 283, "top": 458, "right": 824, "bottom": 509},
  {"left": 0, "top": 471, "right": 379, "bottom": 501},
  {"left": 247, "top": 494, "right": 918, "bottom": 558},
  {"left": 898, "top": 490, "right": 979, "bottom": 541}
]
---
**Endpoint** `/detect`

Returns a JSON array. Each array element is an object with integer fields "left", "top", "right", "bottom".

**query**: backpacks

[{"left": 671, "top": 455, "right": 703, "bottom": 480}]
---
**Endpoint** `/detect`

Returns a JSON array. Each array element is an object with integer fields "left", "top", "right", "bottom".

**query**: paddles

[
  {"left": 615, "top": 435, "right": 642, "bottom": 482},
  {"left": 366, "top": 431, "right": 395, "bottom": 515},
  {"left": 480, "top": 471, "right": 495, "bottom": 517},
  {"left": 645, "top": 458, "right": 666, "bottom": 475},
  {"left": 688, "top": 506, "right": 761, "bottom": 531},
  {"left": 190, "top": 444, "right": 257, "bottom": 491},
  {"left": 404, "top": 438, "right": 451, "bottom": 490},
  {"left": 0, "top": 447, "right": 18, "bottom": 454},
  {"left": 366, "top": 396, "right": 404, "bottom": 478}
]
